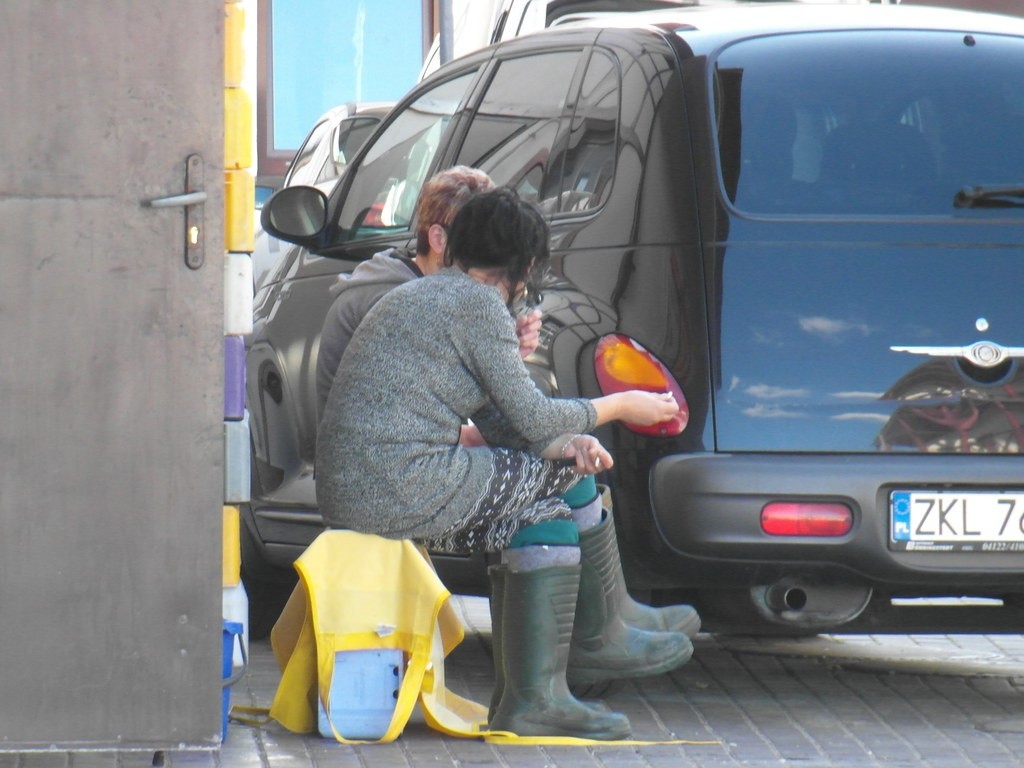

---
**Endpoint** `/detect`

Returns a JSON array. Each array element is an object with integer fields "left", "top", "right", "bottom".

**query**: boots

[
  {"left": 567, "top": 506, "right": 692, "bottom": 683},
  {"left": 489, "top": 565, "right": 632, "bottom": 740},
  {"left": 596, "top": 484, "right": 701, "bottom": 638},
  {"left": 488, "top": 564, "right": 607, "bottom": 724}
]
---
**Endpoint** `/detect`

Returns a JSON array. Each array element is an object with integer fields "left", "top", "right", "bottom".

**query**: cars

[
  {"left": 238, "top": 0, "right": 1024, "bottom": 701},
  {"left": 250, "top": 101, "right": 440, "bottom": 295},
  {"left": 419, "top": 1, "right": 1023, "bottom": 105}
]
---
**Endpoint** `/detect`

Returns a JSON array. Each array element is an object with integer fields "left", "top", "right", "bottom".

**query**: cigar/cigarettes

[
  {"left": 594, "top": 455, "right": 600, "bottom": 468},
  {"left": 665, "top": 390, "right": 673, "bottom": 400},
  {"left": 313, "top": 163, "right": 703, "bottom": 742}
]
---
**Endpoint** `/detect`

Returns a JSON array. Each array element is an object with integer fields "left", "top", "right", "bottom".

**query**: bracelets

[{"left": 561, "top": 433, "right": 582, "bottom": 460}]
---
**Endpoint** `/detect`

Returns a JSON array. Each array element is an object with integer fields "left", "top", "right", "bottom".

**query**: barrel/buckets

[{"left": 222, "top": 619, "right": 247, "bottom": 743}]
